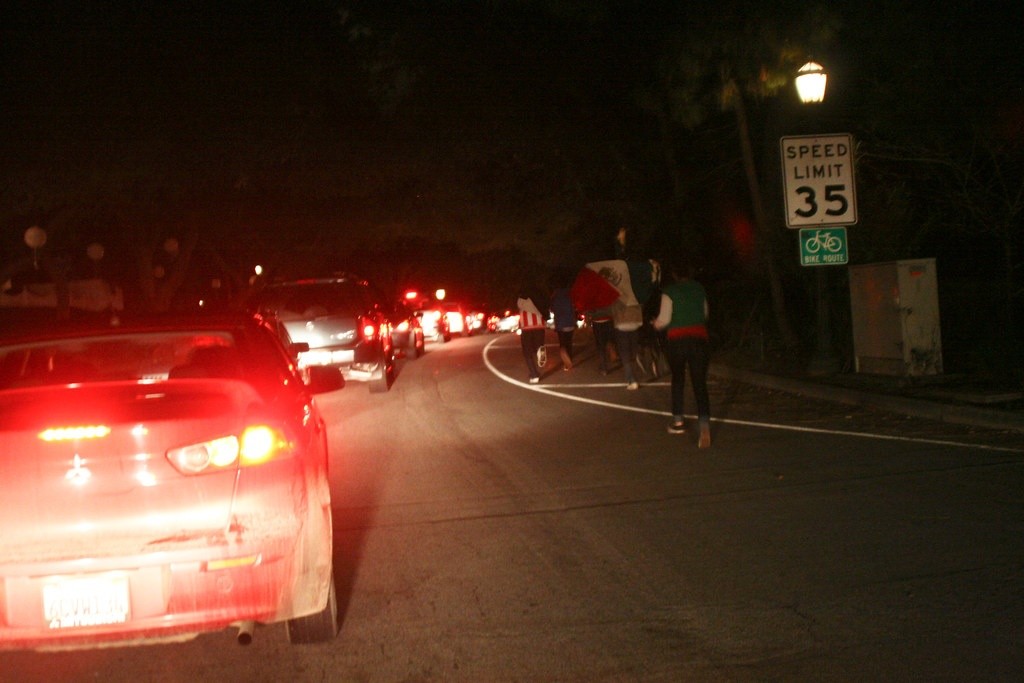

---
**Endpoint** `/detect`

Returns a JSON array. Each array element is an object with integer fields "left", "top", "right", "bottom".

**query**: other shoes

[
  {"left": 528, "top": 373, "right": 540, "bottom": 385},
  {"left": 560, "top": 364, "right": 575, "bottom": 372},
  {"left": 598, "top": 369, "right": 610, "bottom": 376},
  {"left": 625, "top": 381, "right": 640, "bottom": 392}
]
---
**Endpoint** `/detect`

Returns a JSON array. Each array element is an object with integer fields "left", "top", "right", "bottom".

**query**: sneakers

[
  {"left": 699, "top": 423, "right": 711, "bottom": 450},
  {"left": 667, "top": 423, "right": 687, "bottom": 435}
]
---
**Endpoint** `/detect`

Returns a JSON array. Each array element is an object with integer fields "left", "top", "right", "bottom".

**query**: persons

[
  {"left": 550, "top": 260, "right": 661, "bottom": 390},
  {"left": 649, "top": 259, "right": 711, "bottom": 450},
  {"left": 518, "top": 282, "right": 551, "bottom": 384}
]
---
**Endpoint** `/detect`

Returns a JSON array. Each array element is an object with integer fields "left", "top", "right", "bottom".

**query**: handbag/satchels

[{"left": 635, "top": 348, "right": 673, "bottom": 383}]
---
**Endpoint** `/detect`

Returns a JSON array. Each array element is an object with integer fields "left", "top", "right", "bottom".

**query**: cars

[
  {"left": 239, "top": 268, "right": 517, "bottom": 394},
  {"left": 0, "top": 323, "right": 345, "bottom": 653}
]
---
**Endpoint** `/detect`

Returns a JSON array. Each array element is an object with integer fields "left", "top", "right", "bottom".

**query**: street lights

[{"left": 790, "top": 62, "right": 832, "bottom": 383}]
